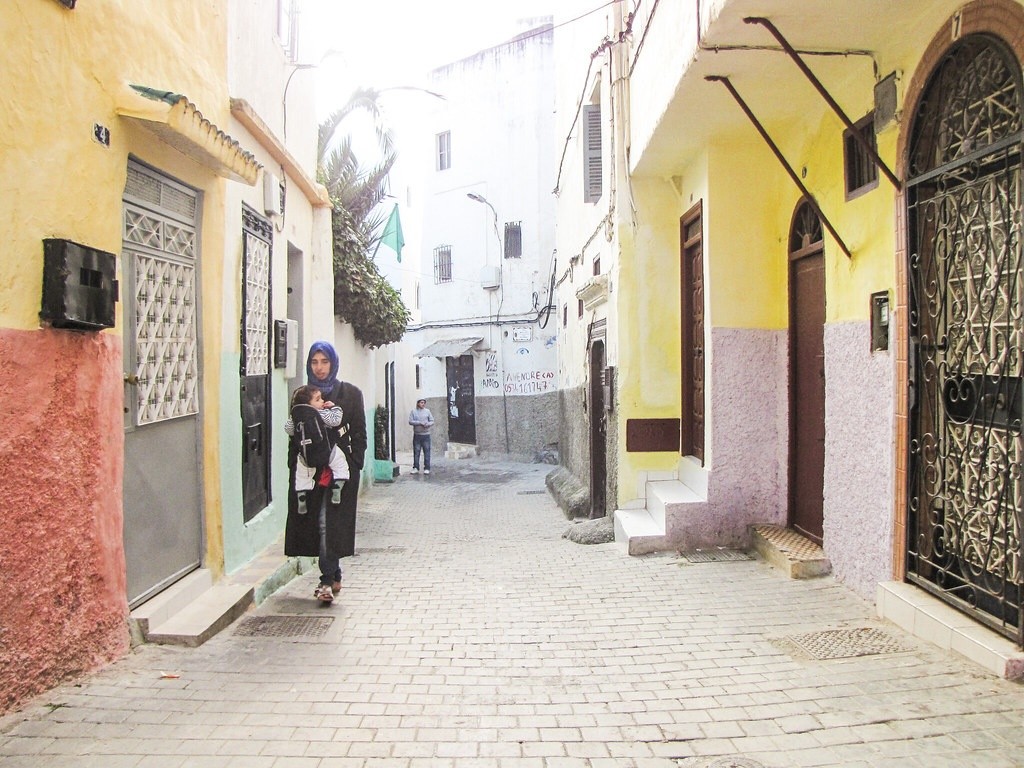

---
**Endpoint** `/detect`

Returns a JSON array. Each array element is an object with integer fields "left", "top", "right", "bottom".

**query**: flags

[{"left": 381, "top": 208, "right": 405, "bottom": 263}]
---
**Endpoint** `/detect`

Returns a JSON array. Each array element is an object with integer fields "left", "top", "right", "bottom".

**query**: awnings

[{"left": 412, "top": 337, "right": 481, "bottom": 359}]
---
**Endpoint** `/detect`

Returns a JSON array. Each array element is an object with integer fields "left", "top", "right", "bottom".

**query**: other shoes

[
  {"left": 410, "top": 468, "right": 419, "bottom": 474},
  {"left": 317, "top": 586, "right": 334, "bottom": 601},
  {"left": 424, "top": 470, "right": 429, "bottom": 474},
  {"left": 314, "top": 580, "right": 341, "bottom": 596}
]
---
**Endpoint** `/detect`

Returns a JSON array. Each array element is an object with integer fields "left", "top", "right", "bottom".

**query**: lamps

[{"left": 467, "top": 192, "right": 498, "bottom": 232}]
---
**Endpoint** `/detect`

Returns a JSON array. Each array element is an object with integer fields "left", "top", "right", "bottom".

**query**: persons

[
  {"left": 409, "top": 399, "right": 434, "bottom": 474},
  {"left": 285, "top": 341, "right": 367, "bottom": 600},
  {"left": 285, "top": 386, "right": 349, "bottom": 514}
]
